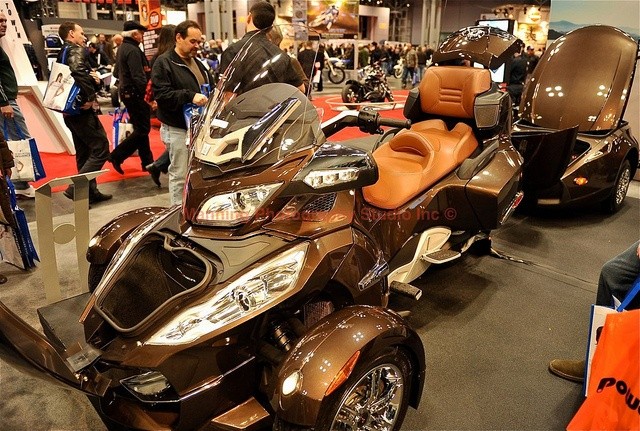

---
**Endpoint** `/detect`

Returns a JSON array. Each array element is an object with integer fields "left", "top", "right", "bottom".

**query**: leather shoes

[
  {"left": 63, "top": 185, "right": 92, "bottom": 209},
  {"left": 106, "top": 155, "right": 124, "bottom": 175},
  {"left": 146, "top": 163, "right": 162, "bottom": 186},
  {"left": 142, "top": 168, "right": 147, "bottom": 171},
  {"left": 550, "top": 358, "right": 587, "bottom": 381},
  {"left": 89, "top": 190, "right": 113, "bottom": 204}
]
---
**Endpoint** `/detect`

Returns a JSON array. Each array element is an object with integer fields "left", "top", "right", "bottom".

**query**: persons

[
  {"left": 508, "top": 42, "right": 545, "bottom": 104},
  {"left": 52, "top": 21, "right": 114, "bottom": 207},
  {"left": 96, "top": 33, "right": 113, "bottom": 66},
  {"left": 106, "top": 19, "right": 165, "bottom": 176},
  {"left": 151, "top": 23, "right": 186, "bottom": 188},
  {"left": 327, "top": 41, "right": 354, "bottom": 67},
  {"left": 0, "top": 12, "right": 41, "bottom": 202},
  {"left": 85, "top": 42, "right": 106, "bottom": 69},
  {"left": 315, "top": 44, "right": 326, "bottom": 94},
  {"left": 266, "top": 23, "right": 309, "bottom": 93},
  {"left": 549, "top": 238, "right": 640, "bottom": 383},
  {"left": 151, "top": 21, "right": 215, "bottom": 205},
  {"left": 215, "top": 2, "right": 305, "bottom": 97},
  {"left": 201, "top": 34, "right": 206, "bottom": 47},
  {"left": 216, "top": 37, "right": 224, "bottom": 54},
  {"left": 208, "top": 39, "right": 220, "bottom": 57},
  {"left": 111, "top": 33, "right": 123, "bottom": 87},
  {"left": 358, "top": 42, "right": 401, "bottom": 78},
  {"left": 0, "top": 127, "right": 15, "bottom": 284},
  {"left": 298, "top": 40, "right": 316, "bottom": 88},
  {"left": 401, "top": 43, "right": 432, "bottom": 85}
]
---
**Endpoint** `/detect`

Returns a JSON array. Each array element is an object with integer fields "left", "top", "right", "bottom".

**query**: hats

[
  {"left": 526, "top": 46, "right": 533, "bottom": 50},
  {"left": 123, "top": 20, "right": 147, "bottom": 31}
]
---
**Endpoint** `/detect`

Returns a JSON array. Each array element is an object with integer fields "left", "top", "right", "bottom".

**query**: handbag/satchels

[
  {"left": 412, "top": 74, "right": 418, "bottom": 84},
  {"left": 0, "top": 204, "right": 40, "bottom": 273},
  {"left": 113, "top": 122, "right": 139, "bottom": 157},
  {"left": 40, "top": 61, "right": 91, "bottom": 114},
  {"left": 184, "top": 94, "right": 210, "bottom": 151},
  {"left": 3, "top": 138, "right": 45, "bottom": 183},
  {"left": 584, "top": 296, "right": 640, "bottom": 399}
]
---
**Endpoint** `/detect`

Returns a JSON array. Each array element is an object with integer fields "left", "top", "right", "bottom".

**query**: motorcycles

[
  {"left": 342, "top": 70, "right": 394, "bottom": 109},
  {"left": 320, "top": 52, "right": 346, "bottom": 83},
  {"left": 392, "top": 55, "right": 406, "bottom": 78},
  {"left": 308, "top": 11, "right": 339, "bottom": 30},
  {"left": 77, "top": 26, "right": 524, "bottom": 431}
]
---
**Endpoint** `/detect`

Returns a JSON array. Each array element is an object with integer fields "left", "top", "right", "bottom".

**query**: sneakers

[
  {"left": 14, "top": 185, "right": 37, "bottom": 201},
  {"left": 0, "top": 274, "right": 8, "bottom": 285},
  {"left": 402, "top": 84, "right": 406, "bottom": 89}
]
